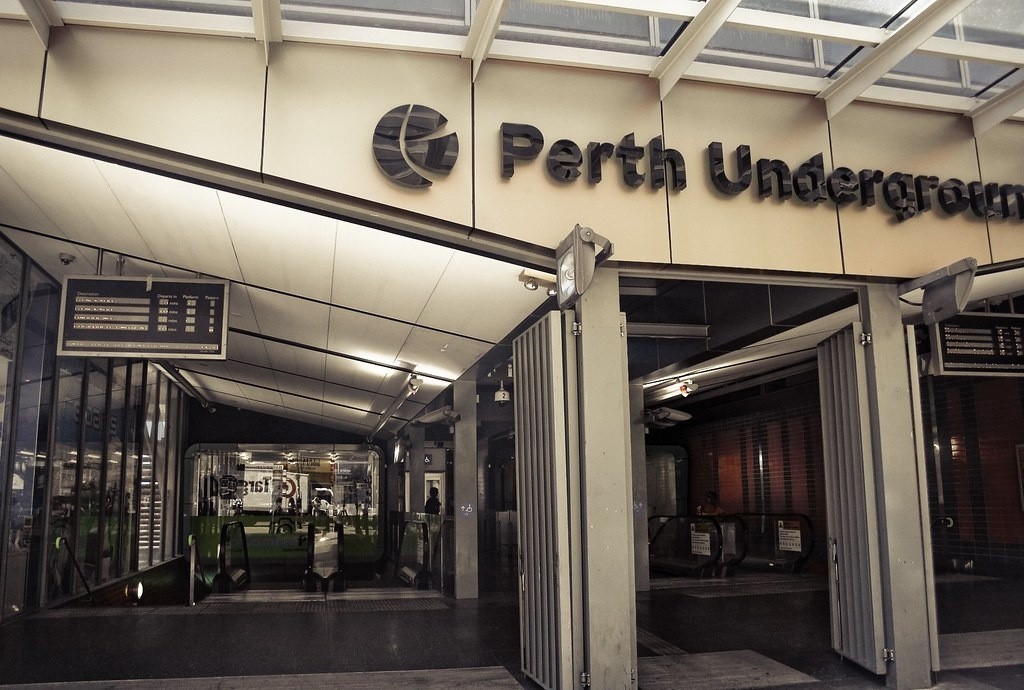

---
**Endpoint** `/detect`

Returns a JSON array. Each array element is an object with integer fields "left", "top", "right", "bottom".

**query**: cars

[{"left": 358, "top": 509, "right": 378, "bottom": 529}]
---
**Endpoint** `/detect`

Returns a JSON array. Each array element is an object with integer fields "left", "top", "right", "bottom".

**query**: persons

[
  {"left": 273, "top": 497, "right": 283, "bottom": 534},
  {"left": 425, "top": 488, "right": 440, "bottom": 513},
  {"left": 287, "top": 497, "right": 299, "bottom": 534},
  {"left": 312, "top": 493, "right": 331, "bottom": 516},
  {"left": 696, "top": 492, "right": 727, "bottom": 517},
  {"left": 11, "top": 497, "right": 25, "bottom": 548}
]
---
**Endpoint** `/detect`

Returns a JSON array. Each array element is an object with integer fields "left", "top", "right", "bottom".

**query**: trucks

[
  {"left": 40, "top": 461, "right": 131, "bottom": 509},
  {"left": 242, "top": 468, "right": 341, "bottom": 520}
]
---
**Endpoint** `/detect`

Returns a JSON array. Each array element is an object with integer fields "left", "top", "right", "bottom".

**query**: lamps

[
  {"left": 394, "top": 437, "right": 410, "bottom": 464},
  {"left": 642, "top": 407, "right": 693, "bottom": 429},
  {"left": 128, "top": 578, "right": 143, "bottom": 604},
  {"left": 644, "top": 379, "right": 698, "bottom": 405},
  {"left": 556, "top": 223, "right": 615, "bottom": 313},
  {"left": 898, "top": 257, "right": 977, "bottom": 325},
  {"left": 366, "top": 373, "right": 423, "bottom": 443},
  {"left": 148, "top": 360, "right": 209, "bottom": 408},
  {"left": 519, "top": 270, "right": 557, "bottom": 296}
]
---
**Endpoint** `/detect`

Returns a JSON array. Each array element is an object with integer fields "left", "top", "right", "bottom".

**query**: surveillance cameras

[
  {"left": 59, "top": 253, "right": 74, "bottom": 265},
  {"left": 494, "top": 390, "right": 510, "bottom": 407}
]
